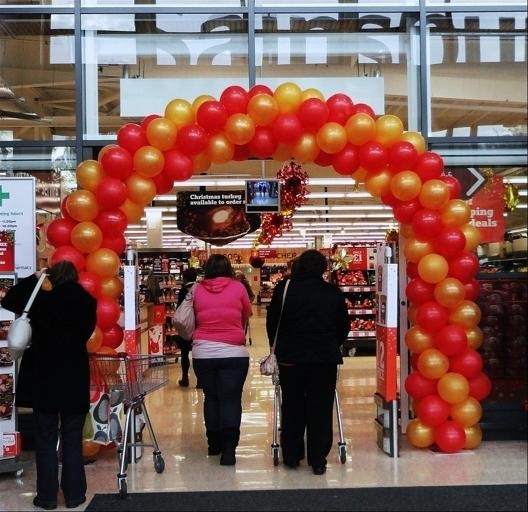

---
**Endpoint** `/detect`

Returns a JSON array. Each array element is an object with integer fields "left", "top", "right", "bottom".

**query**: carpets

[{"left": 84, "top": 483, "right": 528, "bottom": 512}]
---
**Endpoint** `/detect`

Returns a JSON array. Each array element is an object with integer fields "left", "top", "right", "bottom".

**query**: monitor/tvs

[{"left": 245, "top": 179, "right": 280, "bottom": 214}]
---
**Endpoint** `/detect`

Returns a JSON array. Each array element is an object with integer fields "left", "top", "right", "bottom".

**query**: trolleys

[
  {"left": 243, "top": 316, "right": 251, "bottom": 346},
  {"left": 267, "top": 361, "right": 352, "bottom": 470},
  {"left": 79, "top": 348, "right": 185, "bottom": 500}
]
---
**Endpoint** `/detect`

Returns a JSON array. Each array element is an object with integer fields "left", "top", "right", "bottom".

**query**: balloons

[
  {"left": 316, "top": 94, "right": 494, "bottom": 451},
  {"left": 95, "top": 83, "right": 333, "bottom": 223},
  {"left": 47, "top": 160, "right": 155, "bottom": 454}
]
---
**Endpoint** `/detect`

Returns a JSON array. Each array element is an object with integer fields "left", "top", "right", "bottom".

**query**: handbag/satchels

[
  {"left": 172, "top": 293, "right": 195, "bottom": 341},
  {"left": 259, "top": 355, "right": 274, "bottom": 375},
  {"left": 8, "top": 316, "right": 32, "bottom": 360}
]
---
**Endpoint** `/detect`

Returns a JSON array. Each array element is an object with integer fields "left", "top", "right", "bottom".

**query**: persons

[
  {"left": 190, "top": 255, "right": 254, "bottom": 467},
  {"left": 0, "top": 260, "right": 100, "bottom": 510},
  {"left": 264, "top": 247, "right": 352, "bottom": 475},
  {"left": 144, "top": 276, "right": 161, "bottom": 305},
  {"left": 235, "top": 270, "right": 254, "bottom": 303},
  {"left": 176, "top": 269, "right": 203, "bottom": 390}
]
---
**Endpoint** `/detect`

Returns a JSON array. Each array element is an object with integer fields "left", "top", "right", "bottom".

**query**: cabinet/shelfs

[
  {"left": 136, "top": 248, "right": 192, "bottom": 356},
  {"left": 331, "top": 247, "right": 377, "bottom": 357}
]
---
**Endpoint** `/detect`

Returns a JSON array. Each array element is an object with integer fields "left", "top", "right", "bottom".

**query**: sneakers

[
  {"left": 66, "top": 496, "right": 86, "bottom": 508},
  {"left": 33, "top": 497, "right": 57, "bottom": 509}
]
